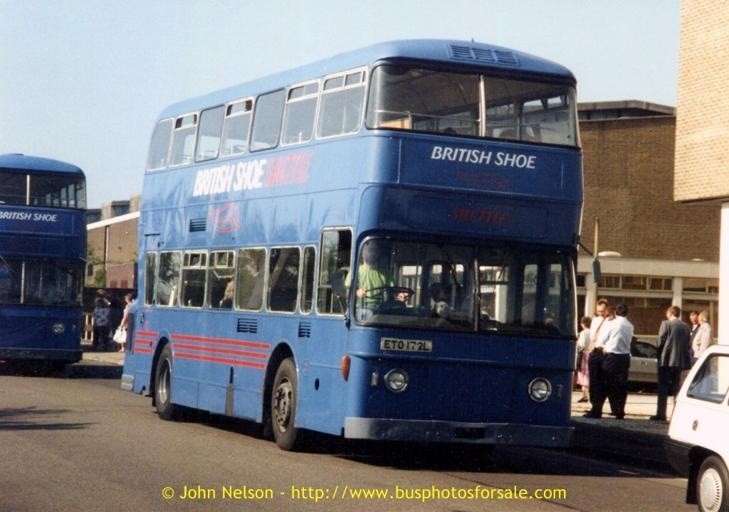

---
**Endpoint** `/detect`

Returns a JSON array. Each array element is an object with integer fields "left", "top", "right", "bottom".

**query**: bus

[
  {"left": 119, "top": 37, "right": 602, "bottom": 452},
  {"left": 0, "top": 153, "right": 88, "bottom": 371}
]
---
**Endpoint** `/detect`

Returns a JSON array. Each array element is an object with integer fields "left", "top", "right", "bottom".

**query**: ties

[{"left": 593, "top": 317, "right": 606, "bottom": 337}]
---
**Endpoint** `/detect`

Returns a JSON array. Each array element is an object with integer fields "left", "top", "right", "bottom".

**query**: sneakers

[{"left": 582, "top": 409, "right": 627, "bottom": 420}]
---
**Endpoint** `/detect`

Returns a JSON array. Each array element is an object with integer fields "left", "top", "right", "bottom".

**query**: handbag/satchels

[
  {"left": 112, "top": 325, "right": 128, "bottom": 344},
  {"left": 577, "top": 350, "right": 584, "bottom": 370}
]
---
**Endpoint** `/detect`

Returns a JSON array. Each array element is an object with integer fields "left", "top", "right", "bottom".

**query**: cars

[
  {"left": 627, "top": 337, "right": 662, "bottom": 385},
  {"left": 663, "top": 345, "right": 729, "bottom": 512}
]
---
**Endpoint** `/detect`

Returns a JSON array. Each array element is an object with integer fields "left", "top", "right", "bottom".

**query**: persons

[
  {"left": 93, "top": 296, "right": 112, "bottom": 351},
  {"left": 425, "top": 281, "right": 451, "bottom": 316},
  {"left": 576, "top": 295, "right": 713, "bottom": 422},
  {"left": 156, "top": 265, "right": 176, "bottom": 305},
  {"left": 394, "top": 290, "right": 413, "bottom": 308},
  {"left": 109, "top": 297, "right": 123, "bottom": 350},
  {"left": 112, "top": 293, "right": 133, "bottom": 353},
  {"left": 338, "top": 235, "right": 411, "bottom": 323},
  {"left": 220, "top": 281, "right": 235, "bottom": 308}
]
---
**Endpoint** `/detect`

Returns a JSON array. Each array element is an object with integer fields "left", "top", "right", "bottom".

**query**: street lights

[{"left": 598, "top": 251, "right": 624, "bottom": 289}]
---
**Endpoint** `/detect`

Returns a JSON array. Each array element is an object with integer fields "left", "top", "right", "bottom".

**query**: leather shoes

[
  {"left": 650, "top": 414, "right": 667, "bottom": 421},
  {"left": 578, "top": 397, "right": 589, "bottom": 403}
]
